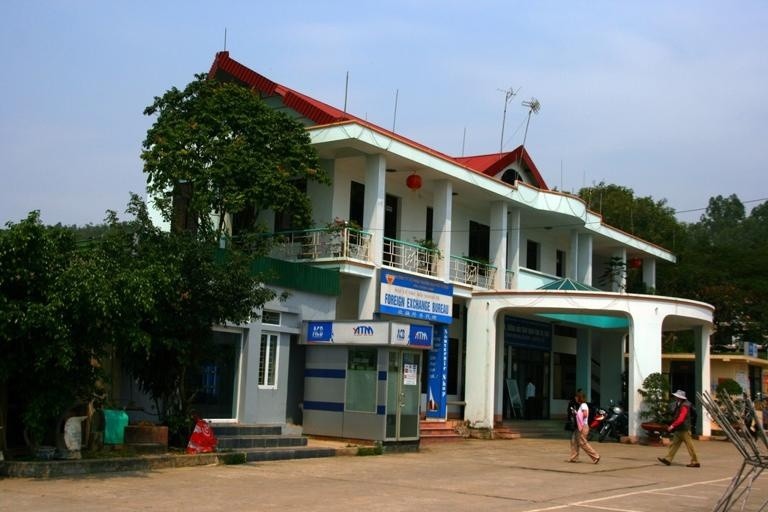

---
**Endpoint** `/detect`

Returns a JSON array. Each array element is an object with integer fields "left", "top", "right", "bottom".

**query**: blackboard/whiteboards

[{"left": 506, "top": 380, "right": 523, "bottom": 408}]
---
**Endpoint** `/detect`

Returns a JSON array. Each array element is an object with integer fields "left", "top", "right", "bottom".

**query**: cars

[{"left": 727, "top": 340, "right": 763, "bottom": 349}]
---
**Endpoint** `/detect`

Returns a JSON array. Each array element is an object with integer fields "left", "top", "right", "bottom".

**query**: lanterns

[
  {"left": 630, "top": 256, "right": 641, "bottom": 270},
  {"left": 407, "top": 174, "right": 423, "bottom": 192}
]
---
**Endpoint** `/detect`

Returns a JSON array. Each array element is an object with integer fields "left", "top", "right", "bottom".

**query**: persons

[
  {"left": 524, "top": 378, "right": 536, "bottom": 420},
  {"left": 568, "top": 388, "right": 582, "bottom": 450},
  {"left": 743, "top": 392, "right": 758, "bottom": 442},
  {"left": 657, "top": 390, "right": 700, "bottom": 467},
  {"left": 565, "top": 392, "right": 600, "bottom": 464}
]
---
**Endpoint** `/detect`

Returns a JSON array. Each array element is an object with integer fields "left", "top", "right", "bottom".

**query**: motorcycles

[
  {"left": 586, "top": 405, "right": 622, "bottom": 442},
  {"left": 597, "top": 396, "right": 628, "bottom": 444}
]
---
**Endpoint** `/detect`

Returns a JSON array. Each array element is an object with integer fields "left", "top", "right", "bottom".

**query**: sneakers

[
  {"left": 564, "top": 459, "right": 577, "bottom": 462},
  {"left": 593, "top": 455, "right": 601, "bottom": 464},
  {"left": 657, "top": 456, "right": 671, "bottom": 466},
  {"left": 686, "top": 463, "right": 700, "bottom": 467}
]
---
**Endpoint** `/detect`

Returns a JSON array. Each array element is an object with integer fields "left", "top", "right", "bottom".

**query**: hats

[{"left": 672, "top": 390, "right": 688, "bottom": 401}]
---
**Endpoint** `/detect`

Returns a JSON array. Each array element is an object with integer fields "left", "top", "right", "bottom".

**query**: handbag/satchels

[{"left": 564, "top": 416, "right": 576, "bottom": 432}]
